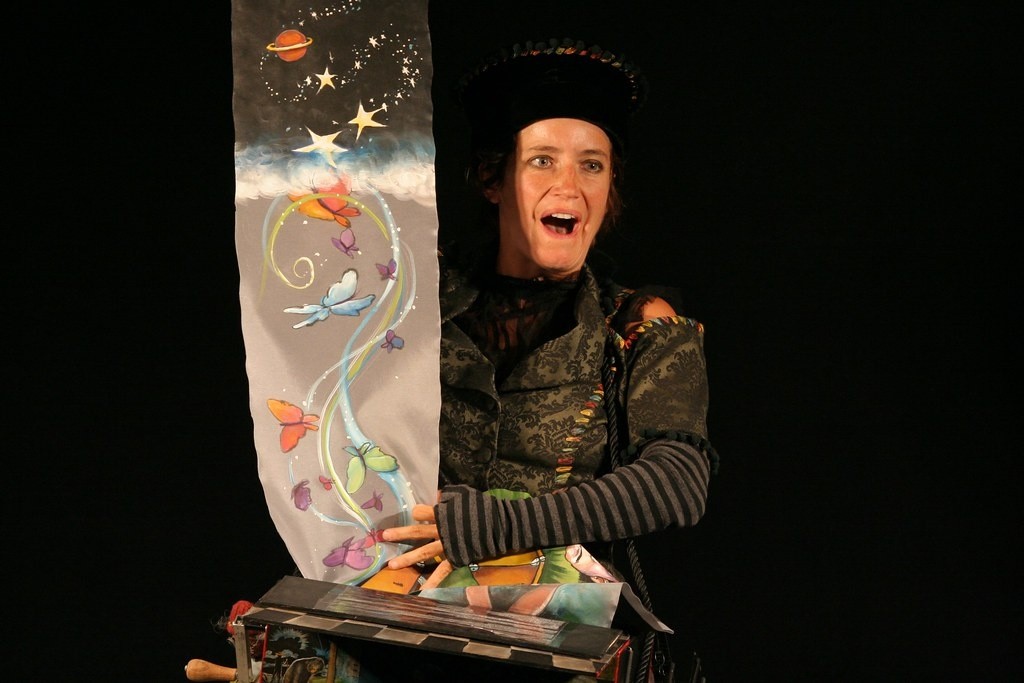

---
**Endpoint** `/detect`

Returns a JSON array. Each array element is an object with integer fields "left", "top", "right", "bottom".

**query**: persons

[{"left": 381, "top": 41, "right": 720, "bottom": 683}]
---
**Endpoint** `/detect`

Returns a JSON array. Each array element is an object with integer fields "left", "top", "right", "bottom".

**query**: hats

[{"left": 470, "top": 43, "right": 638, "bottom": 147}]
